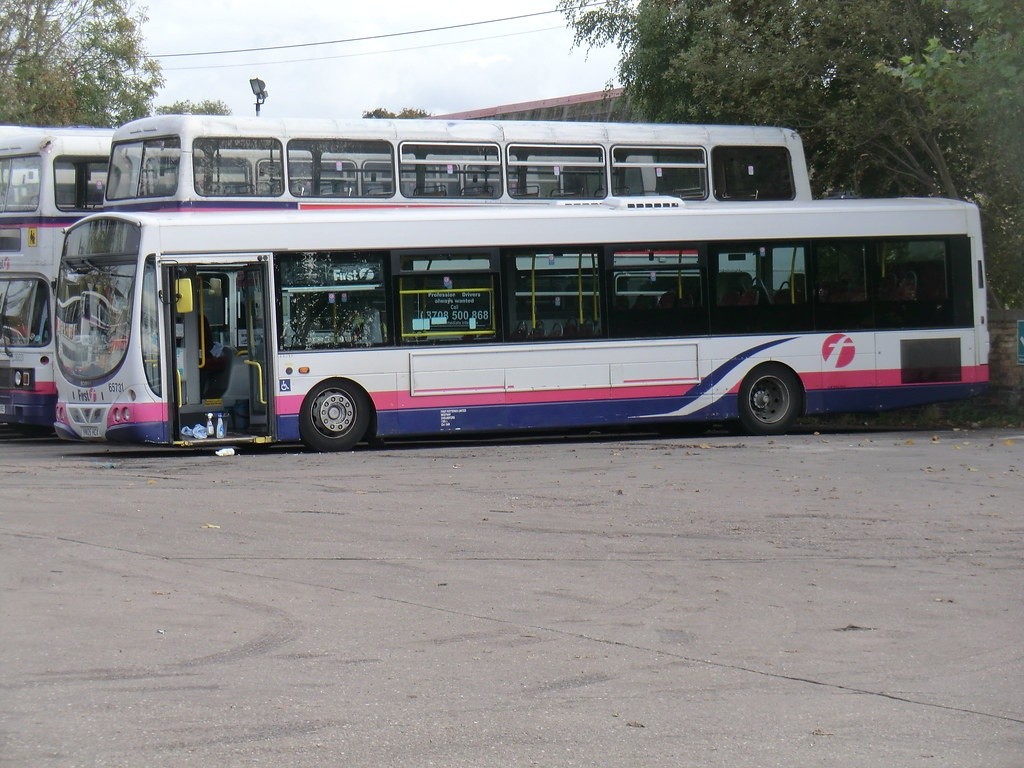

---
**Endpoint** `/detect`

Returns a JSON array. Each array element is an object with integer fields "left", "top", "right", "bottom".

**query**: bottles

[{"left": 217, "top": 412, "right": 225, "bottom": 438}]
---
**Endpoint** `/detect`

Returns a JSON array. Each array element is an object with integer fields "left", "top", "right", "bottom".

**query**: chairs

[
  {"left": 197, "top": 313, "right": 227, "bottom": 394},
  {"left": 459, "top": 266, "right": 917, "bottom": 343}
]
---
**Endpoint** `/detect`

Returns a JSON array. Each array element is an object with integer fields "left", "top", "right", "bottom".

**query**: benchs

[{"left": 224, "top": 184, "right": 761, "bottom": 201}]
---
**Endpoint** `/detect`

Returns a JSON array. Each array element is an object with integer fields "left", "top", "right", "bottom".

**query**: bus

[{"left": 0, "top": 113, "right": 991, "bottom": 453}]
